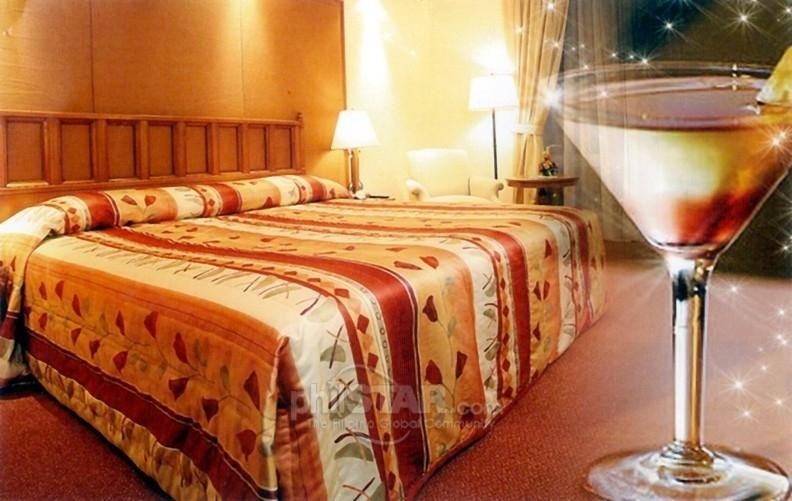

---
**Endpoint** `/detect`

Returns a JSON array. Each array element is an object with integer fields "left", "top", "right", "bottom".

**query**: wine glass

[{"left": 532, "top": 62, "right": 790, "bottom": 501}]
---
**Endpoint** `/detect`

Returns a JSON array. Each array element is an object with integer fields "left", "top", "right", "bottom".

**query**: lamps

[
  {"left": 331, "top": 105, "right": 379, "bottom": 198},
  {"left": 467, "top": 71, "right": 518, "bottom": 180}
]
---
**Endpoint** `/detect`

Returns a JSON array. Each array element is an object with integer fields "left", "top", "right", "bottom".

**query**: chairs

[{"left": 404, "top": 148, "right": 508, "bottom": 207}]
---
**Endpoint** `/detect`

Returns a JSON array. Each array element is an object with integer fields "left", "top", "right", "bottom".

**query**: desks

[{"left": 506, "top": 174, "right": 579, "bottom": 204}]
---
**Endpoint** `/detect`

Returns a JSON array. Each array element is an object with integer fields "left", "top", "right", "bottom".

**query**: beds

[{"left": 0, "top": 109, "right": 610, "bottom": 499}]
